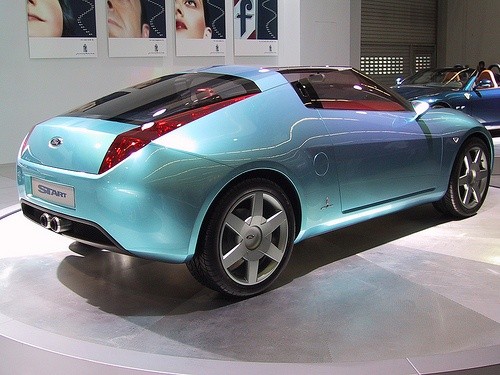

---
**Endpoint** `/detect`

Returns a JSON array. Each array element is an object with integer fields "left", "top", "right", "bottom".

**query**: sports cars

[{"left": 387, "top": 62, "right": 500, "bottom": 131}]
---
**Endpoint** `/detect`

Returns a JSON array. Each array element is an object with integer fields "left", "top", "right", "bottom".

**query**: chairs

[
  {"left": 441, "top": 72, "right": 460, "bottom": 85},
  {"left": 472, "top": 70, "right": 498, "bottom": 89}
]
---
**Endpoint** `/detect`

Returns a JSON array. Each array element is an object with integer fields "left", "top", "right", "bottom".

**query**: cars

[{"left": 15, "top": 64, "right": 495, "bottom": 298}]
